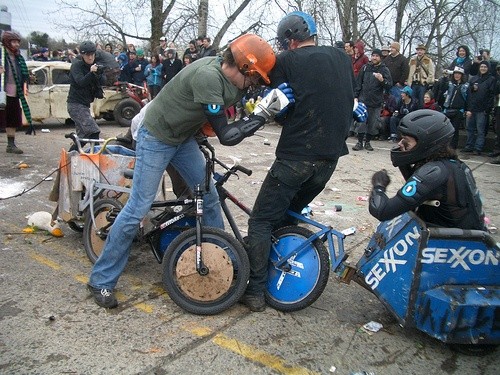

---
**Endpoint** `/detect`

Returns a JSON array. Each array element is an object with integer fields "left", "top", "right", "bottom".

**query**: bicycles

[
  {"left": 77, "top": 145, "right": 250, "bottom": 316},
  {"left": 64, "top": 131, "right": 132, "bottom": 233},
  {"left": 201, "top": 140, "right": 350, "bottom": 313}
]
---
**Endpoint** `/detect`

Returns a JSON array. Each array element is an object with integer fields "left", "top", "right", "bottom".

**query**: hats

[
  {"left": 454, "top": 66, "right": 465, "bottom": 74},
  {"left": 380, "top": 46, "right": 389, "bottom": 51},
  {"left": 372, "top": 49, "right": 383, "bottom": 58},
  {"left": 399, "top": 86, "right": 413, "bottom": 97},
  {"left": 41, "top": 47, "right": 49, "bottom": 53},
  {"left": 480, "top": 60, "right": 491, "bottom": 68},
  {"left": 415, "top": 44, "right": 426, "bottom": 51},
  {"left": 158, "top": 37, "right": 166, "bottom": 41},
  {"left": 166, "top": 49, "right": 176, "bottom": 58},
  {"left": 136, "top": 49, "right": 144, "bottom": 55},
  {"left": 390, "top": 43, "right": 400, "bottom": 52},
  {"left": 196, "top": 36, "right": 203, "bottom": 39}
]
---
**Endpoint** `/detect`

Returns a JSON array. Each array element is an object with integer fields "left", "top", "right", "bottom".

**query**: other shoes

[
  {"left": 473, "top": 149, "right": 480, "bottom": 155},
  {"left": 461, "top": 148, "right": 471, "bottom": 152},
  {"left": 365, "top": 141, "right": 374, "bottom": 151},
  {"left": 241, "top": 292, "right": 266, "bottom": 311},
  {"left": 491, "top": 159, "right": 500, "bottom": 164},
  {"left": 353, "top": 143, "right": 363, "bottom": 150},
  {"left": 488, "top": 151, "right": 499, "bottom": 157},
  {"left": 6, "top": 145, "right": 23, "bottom": 153}
]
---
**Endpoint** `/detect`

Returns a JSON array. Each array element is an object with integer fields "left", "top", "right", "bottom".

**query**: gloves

[
  {"left": 372, "top": 168, "right": 390, "bottom": 190},
  {"left": 352, "top": 99, "right": 368, "bottom": 123},
  {"left": 255, "top": 82, "right": 296, "bottom": 119}
]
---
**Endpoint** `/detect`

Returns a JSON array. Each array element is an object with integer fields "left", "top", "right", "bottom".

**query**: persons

[
  {"left": 367, "top": 110, "right": 488, "bottom": 233},
  {"left": 237, "top": 11, "right": 367, "bottom": 311},
  {"left": 0, "top": 24, "right": 500, "bottom": 163},
  {"left": 85, "top": 33, "right": 295, "bottom": 308}
]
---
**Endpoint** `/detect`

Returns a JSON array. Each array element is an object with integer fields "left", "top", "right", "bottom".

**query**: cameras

[{"left": 477, "top": 48, "right": 485, "bottom": 61}]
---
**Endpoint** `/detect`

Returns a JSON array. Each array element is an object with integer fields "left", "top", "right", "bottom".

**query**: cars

[{"left": 0, "top": 60, "right": 152, "bottom": 133}]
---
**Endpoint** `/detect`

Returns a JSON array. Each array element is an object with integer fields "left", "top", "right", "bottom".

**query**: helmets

[
  {"left": 229, "top": 34, "right": 276, "bottom": 84},
  {"left": 79, "top": 41, "right": 97, "bottom": 53},
  {"left": 389, "top": 110, "right": 456, "bottom": 168},
  {"left": 277, "top": 12, "right": 317, "bottom": 47},
  {"left": 2, "top": 31, "right": 21, "bottom": 52}
]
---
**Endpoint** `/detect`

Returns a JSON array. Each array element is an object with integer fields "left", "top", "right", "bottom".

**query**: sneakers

[{"left": 89, "top": 283, "right": 117, "bottom": 308}]
